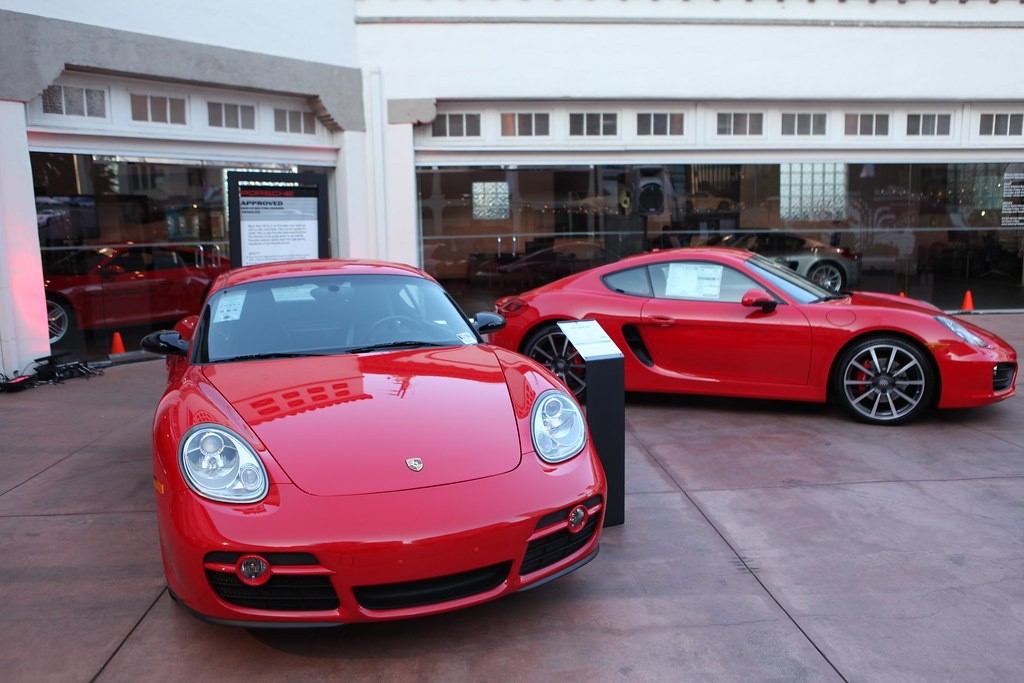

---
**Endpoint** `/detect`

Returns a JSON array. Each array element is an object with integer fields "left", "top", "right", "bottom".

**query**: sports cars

[
  {"left": 484, "top": 247, "right": 1018, "bottom": 428},
  {"left": 42, "top": 242, "right": 231, "bottom": 347},
  {"left": 140, "top": 256, "right": 606, "bottom": 628}
]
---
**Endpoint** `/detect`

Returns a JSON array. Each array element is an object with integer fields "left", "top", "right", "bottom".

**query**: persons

[{"left": 653, "top": 223, "right": 692, "bottom": 250}]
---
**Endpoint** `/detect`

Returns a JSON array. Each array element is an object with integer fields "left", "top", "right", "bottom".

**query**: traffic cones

[
  {"left": 112, "top": 332, "right": 127, "bottom": 355},
  {"left": 897, "top": 293, "right": 905, "bottom": 297},
  {"left": 961, "top": 290, "right": 974, "bottom": 312}
]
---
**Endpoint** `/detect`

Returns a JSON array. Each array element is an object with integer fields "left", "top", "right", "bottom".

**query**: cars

[
  {"left": 477, "top": 241, "right": 626, "bottom": 293},
  {"left": 689, "top": 228, "right": 861, "bottom": 295}
]
